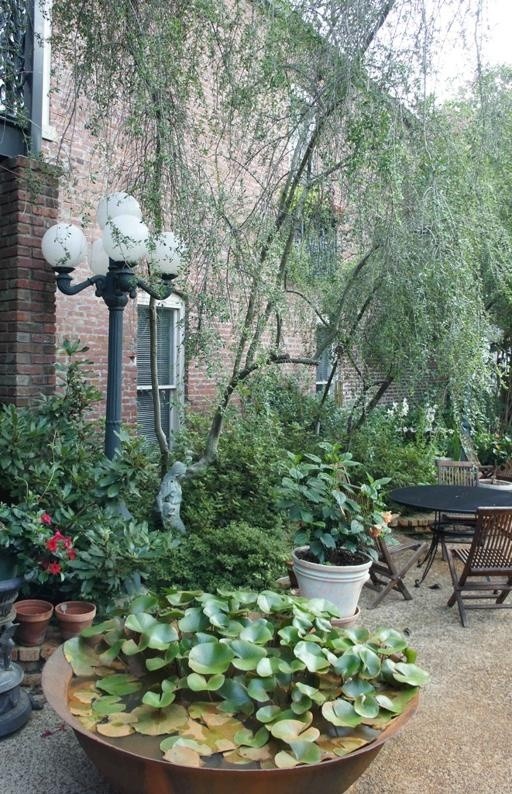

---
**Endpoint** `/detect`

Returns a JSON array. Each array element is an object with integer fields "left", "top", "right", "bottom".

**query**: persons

[{"left": 156, "top": 461, "right": 186, "bottom": 534}]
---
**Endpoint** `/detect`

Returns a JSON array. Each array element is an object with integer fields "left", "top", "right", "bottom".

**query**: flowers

[{"left": 278, "top": 439, "right": 400, "bottom": 564}]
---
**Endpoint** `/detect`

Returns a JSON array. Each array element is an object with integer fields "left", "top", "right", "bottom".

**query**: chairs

[
  {"left": 336, "top": 482, "right": 428, "bottom": 609},
  {"left": 436, "top": 461, "right": 512, "bottom": 628}
]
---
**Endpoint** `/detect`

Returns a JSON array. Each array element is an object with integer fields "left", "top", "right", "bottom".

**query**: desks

[{"left": 387, "top": 486, "right": 512, "bottom": 587}]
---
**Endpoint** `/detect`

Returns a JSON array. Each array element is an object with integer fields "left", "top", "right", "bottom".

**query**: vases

[
  {"left": 291, "top": 546, "right": 372, "bottom": 619},
  {"left": 13, "top": 599, "right": 97, "bottom": 646}
]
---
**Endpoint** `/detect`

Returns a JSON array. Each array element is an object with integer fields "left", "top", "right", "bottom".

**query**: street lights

[{"left": 42, "top": 191, "right": 184, "bottom": 496}]
